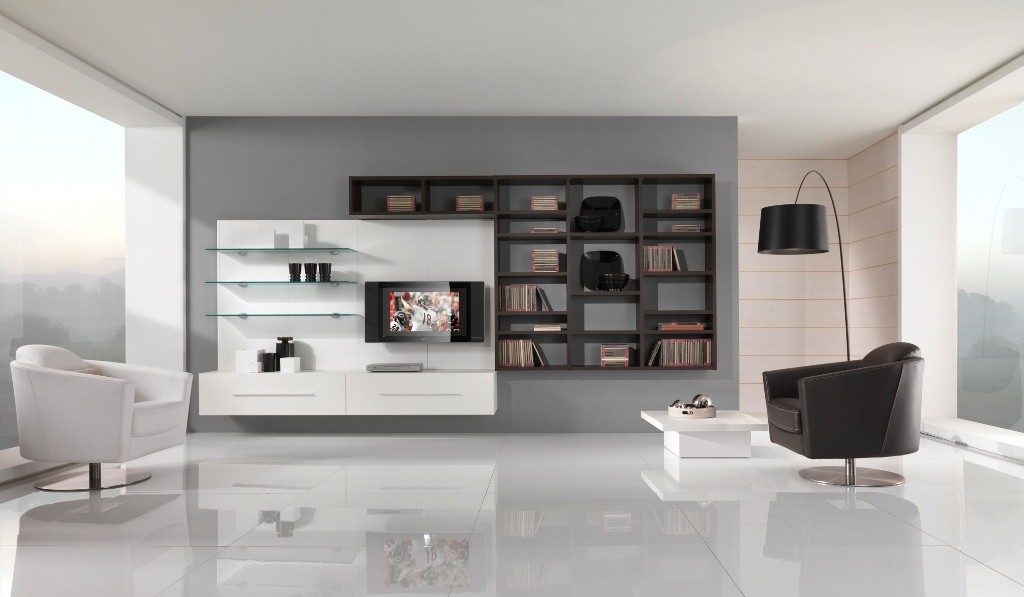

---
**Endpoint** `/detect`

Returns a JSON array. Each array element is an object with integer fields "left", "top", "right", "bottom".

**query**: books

[
  {"left": 671, "top": 225, "right": 701, "bottom": 232},
  {"left": 530, "top": 228, "right": 558, "bottom": 233},
  {"left": 387, "top": 196, "right": 416, "bottom": 212},
  {"left": 499, "top": 286, "right": 551, "bottom": 311},
  {"left": 672, "top": 193, "right": 701, "bottom": 210},
  {"left": 456, "top": 195, "right": 484, "bottom": 211},
  {"left": 643, "top": 246, "right": 682, "bottom": 272},
  {"left": 600, "top": 344, "right": 629, "bottom": 367},
  {"left": 498, "top": 340, "right": 545, "bottom": 367},
  {"left": 657, "top": 321, "right": 706, "bottom": 330},
  {"left": 531, "top": 196, "right": 558, "bottom": 210},
  {"left": 532, "top": 249, "right": 559, "bottom": 273},
  {"left": 648, "top": 338, "right": 711, "bottom": 367},
  {"left": 534, "top": 325, "right": 561, "bottom": 332}
]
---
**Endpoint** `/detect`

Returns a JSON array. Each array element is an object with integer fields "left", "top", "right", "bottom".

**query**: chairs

[{"left": 762, "top": 342, "right": 924, "bottom": 487}]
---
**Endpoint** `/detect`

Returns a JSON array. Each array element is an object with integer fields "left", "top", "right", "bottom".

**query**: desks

[{"left": 640, "top": 410, "right": 778, "bottom": 502}]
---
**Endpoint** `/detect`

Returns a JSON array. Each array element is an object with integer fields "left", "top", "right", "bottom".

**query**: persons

[{"left": 391, "top": 292, "right": 459, "bottom": 332}]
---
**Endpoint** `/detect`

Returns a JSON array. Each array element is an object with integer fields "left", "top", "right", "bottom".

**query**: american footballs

[{"left": 402, "top": 292, "right": 411, "bottom": 301}]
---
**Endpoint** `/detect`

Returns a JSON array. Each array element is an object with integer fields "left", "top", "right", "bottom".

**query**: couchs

[{"left": 10, "top": 343, "right": 193, "bottom": 492}]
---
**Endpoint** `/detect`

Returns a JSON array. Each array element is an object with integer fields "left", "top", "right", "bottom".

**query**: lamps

[{"left": 757, "top": 172, "right": 851, "bottom": 364}]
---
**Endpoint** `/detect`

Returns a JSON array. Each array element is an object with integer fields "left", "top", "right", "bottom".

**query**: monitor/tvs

[{"left": 365, "top": 281, "right": 485, "bottom": 343}]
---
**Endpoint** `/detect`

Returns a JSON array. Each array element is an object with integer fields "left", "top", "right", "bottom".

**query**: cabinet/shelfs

[
  {"left": 204, "top": 248, "right": 357, "bottom": 318},
  {"left": 349, "top": 175, "right": 718, "bottom": 370}
]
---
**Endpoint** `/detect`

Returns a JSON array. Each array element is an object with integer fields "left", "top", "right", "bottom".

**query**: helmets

[
  {"left": 394, "top": 312, "right": 406, "bottom": 327},
  {"left": 418, "top": 294, "right": 431, "bottom": 310}
]
